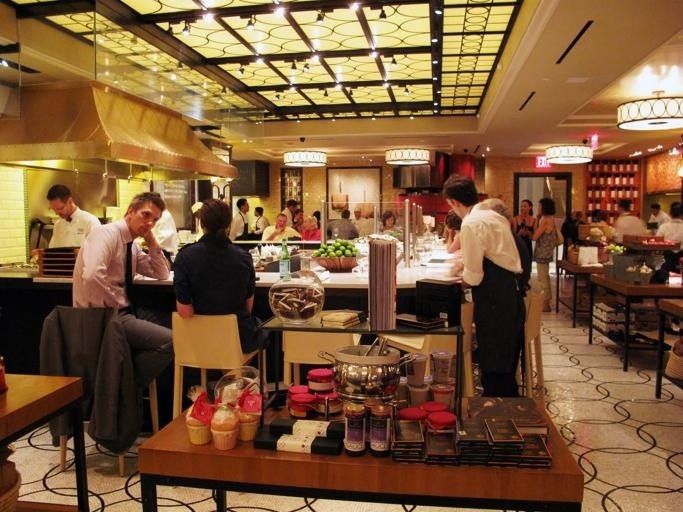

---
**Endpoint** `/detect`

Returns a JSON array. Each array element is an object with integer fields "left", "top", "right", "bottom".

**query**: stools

[
  {"left": 461, "top": 301, "right": 533, "bottom": 396},
  {"left": 381, "top": 334, "right": 457, "bottom": 376},
  {"left": 57, "top": 306, "right": 159, "bottom": 477},
  {"left": 281, "top": 330, "right": 362, "bottom": 386},
  {"left": 173, "top": 312, "right": 267, "bottom": 422},
  {"left": 523, "top": 289, "right": 546, "bottom": 388}
]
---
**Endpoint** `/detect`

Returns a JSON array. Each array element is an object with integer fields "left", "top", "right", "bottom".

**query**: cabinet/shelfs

[{"left": 585, "top": 157, "right": 646, "bottom": 216}]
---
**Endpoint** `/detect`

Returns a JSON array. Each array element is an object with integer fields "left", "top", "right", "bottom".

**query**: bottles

[{"left": 279, "top": 238, "right": 290, "bottom": 279}]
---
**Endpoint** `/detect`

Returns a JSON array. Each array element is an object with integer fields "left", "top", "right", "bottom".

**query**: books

[{"left": 466, "top": 397, "right": 548, "bottom": 445}]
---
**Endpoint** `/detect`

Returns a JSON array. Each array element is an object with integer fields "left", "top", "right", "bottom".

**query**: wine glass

[{"left": 356, "top": 251, "right": 369, "bottom": 280}]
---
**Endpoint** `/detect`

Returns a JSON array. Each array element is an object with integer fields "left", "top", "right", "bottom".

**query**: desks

[
  {"left": 138, "top": 397, "right": 584, "bottom": 512},
  {"left": 556, "top": 260, "right": 683, "bottom": 399},
  {"left": 0, "top": 375, "right": 90, "bottom": 512}
]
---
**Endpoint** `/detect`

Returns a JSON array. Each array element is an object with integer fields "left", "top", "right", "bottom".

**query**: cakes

[{"left": 186, "top": 404, "right": 261, "bottom": 450}]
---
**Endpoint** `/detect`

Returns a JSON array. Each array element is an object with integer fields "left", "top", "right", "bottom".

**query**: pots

[{"left": 317, "top": 344, "right": 416, "bottom": 400}]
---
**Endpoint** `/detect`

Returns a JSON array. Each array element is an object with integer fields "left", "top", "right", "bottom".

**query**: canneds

[{"left": 287, "top": 368, "right": 456, "bottom": 458}]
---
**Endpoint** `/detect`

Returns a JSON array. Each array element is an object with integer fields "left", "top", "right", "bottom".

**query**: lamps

[
  {"left": 183, "top": 0, "right": 442, "bottom": 124},
  {"left": 284, "top": 152, "right": 327, "bottom": 167},
  {"left": 545, "top": 143, "right": 593, "bottom": 164},
  {"left": 385, "top": 148, "right": 431, "bottom": 166},
  {"left": 616, "top": 91, "right": 683, "bottom": 131}
]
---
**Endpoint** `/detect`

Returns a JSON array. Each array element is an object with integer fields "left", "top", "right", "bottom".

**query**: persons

[
  {"left": 378, "top": 211, "right": 395, "bottom": 233},
  {"left": 191, "top": 199, "right": 359, "bottom": 241},
  {"left": 138, "top": 193, "right": 174, "bottom": 271},
  {"left": 441, "top": 198, "right": 683, "bottom": 313},
  {"left": 31, "top": 185, "right": 101, "bottom": 258},
  {"left": 173, "top": 199, "right": 273, "bottom": 383},
  {"left": 72, "top": 193, "right": 175, "bottom": 386},
  {"left": 443, "top": 174, "right": 526, "bottom": 398}
]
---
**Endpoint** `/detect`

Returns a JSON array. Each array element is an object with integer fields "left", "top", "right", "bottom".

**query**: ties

[{"left": 126, "top": 241, "right": 137, "bottom": 318}]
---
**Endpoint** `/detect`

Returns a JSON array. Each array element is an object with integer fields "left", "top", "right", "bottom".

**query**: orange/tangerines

[
  {"left": 608, "top": 244, "right": 627, "bottom": 254},
  {"left": 311, "top": 238, "right": 361, "bottom": 258}
]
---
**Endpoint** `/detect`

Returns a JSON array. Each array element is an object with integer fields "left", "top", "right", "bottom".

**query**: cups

[
  {"left": 397, "top": 352, "right": 455, "bottom": 411},
  {"left": 300, "top": 257, "right": 310, "bottom": 272}
]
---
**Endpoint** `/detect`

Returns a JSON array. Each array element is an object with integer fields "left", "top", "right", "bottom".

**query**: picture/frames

[{"left": 325, "top": 165, "right": 382, "bottom": 221}]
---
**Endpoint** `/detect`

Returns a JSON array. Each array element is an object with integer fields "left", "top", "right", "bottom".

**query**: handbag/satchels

[{"left": 551, "top": 215, "right": 564, "bottom": 245}]
